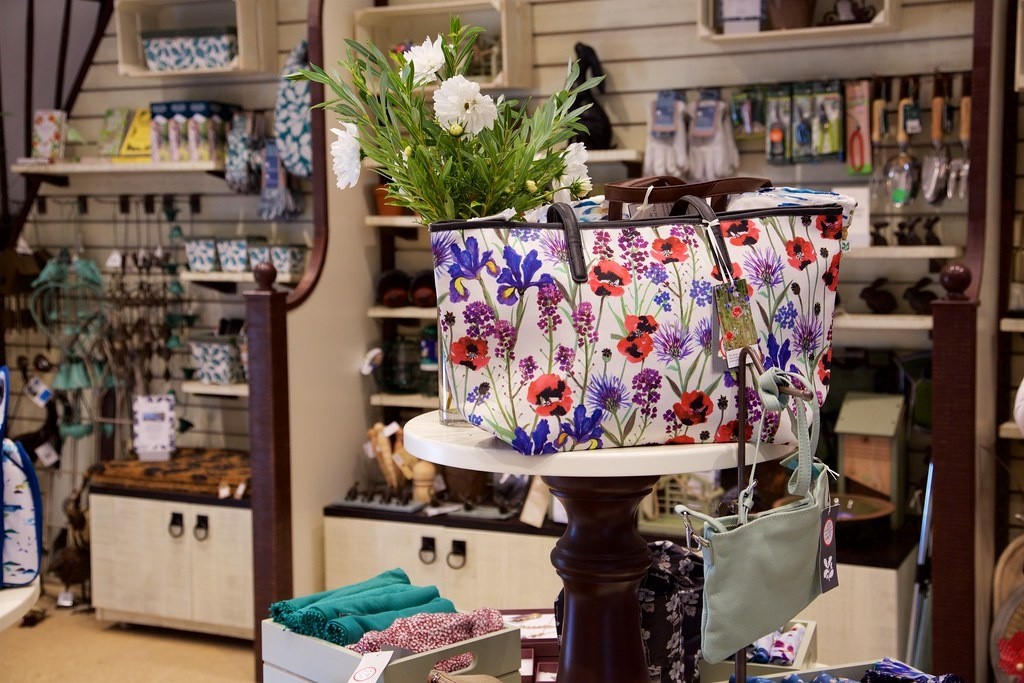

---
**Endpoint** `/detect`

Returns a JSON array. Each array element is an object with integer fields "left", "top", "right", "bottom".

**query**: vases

[
  {"left": 437, "top": 312, "right": 477, "bottom": 427},
  {"left": 139, "top": 25, "right": 238, "bottom": 73},
  {"left": 184, "top": 236, "right": 306, "bottom": 274},
  {"left": 190, "top": 332, "right": 247, "bottom": 385},
  {"left": 372, "top": 184, "right": 405, "bottom": 214}
]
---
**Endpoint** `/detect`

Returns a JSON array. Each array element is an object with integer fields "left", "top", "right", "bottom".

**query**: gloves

[
  {"left": 687, "top": 101, "right": 740, "bottom": 181},
  {"left": 642, "top": 98, "right": 692, "bottom": 178},
  {"left": 224, "top": 129, "right": 257, "bottom": 192},
  {"left": 255, "top": 144, "right": 304, "bottom": 223}
]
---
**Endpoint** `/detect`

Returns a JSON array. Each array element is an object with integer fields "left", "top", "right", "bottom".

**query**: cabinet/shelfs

[
  {"left": 244, "top": 0, "right": 1000, "bottom": 683},
  {"left": 12, "top": 0, "right": 310, "bottom": 642}
]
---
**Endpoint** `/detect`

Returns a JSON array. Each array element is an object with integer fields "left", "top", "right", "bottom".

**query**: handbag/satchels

[
  {"left": 428, "top": 171, "right": 860, "bottom": 456},
  {"left": 675, "top": 365, "right": 841, "bottom": 659}
]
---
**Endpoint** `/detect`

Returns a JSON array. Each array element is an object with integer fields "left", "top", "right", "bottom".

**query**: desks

[{"left": 404, "top": 409, "right": 795, "bottom": 683}]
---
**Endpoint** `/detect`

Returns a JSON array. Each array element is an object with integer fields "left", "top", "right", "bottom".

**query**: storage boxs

[
  {"left": 261, "top": 608, "right": 926, "bottom": 683},
  {"left": 151, "top": 100, "right": 243, "bottom": 161}
]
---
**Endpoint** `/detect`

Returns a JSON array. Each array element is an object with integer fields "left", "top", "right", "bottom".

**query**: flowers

[{"left": 280, "top": 12, "right": 607, "bottom": 225}]
